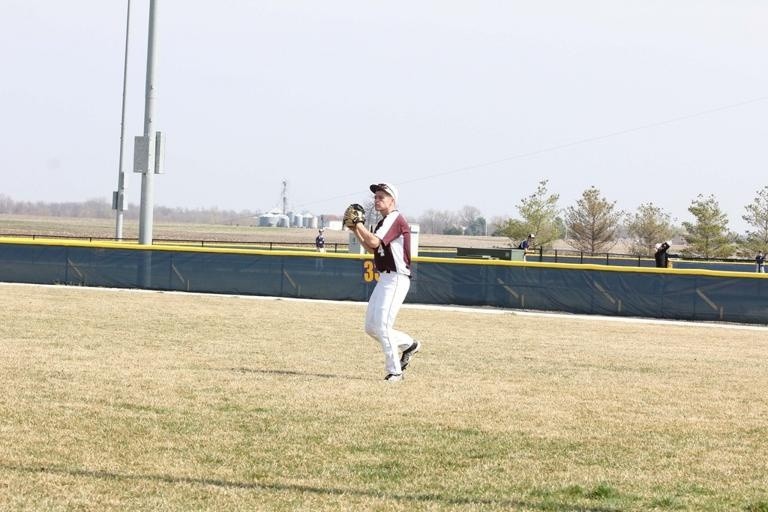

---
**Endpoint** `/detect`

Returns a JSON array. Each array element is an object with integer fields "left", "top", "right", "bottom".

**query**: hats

[
  {"left": 527, "top": 233, "right": 536, "bottom": 238},
  {"left": 318, "top": 229, "right": 325, "bottom": 233},
  {"left": 370, "top": 183, "right": 398, "bottom": 200}
]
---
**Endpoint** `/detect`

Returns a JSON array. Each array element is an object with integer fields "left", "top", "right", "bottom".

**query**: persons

[
  {"left": 519, "top": 233, "right": 535, "bottom": 261},
  {"left": 345, "top": 183, "right": 421, "bottom": 381},
  {"left": 756, "top": 250, "right": 766, "bottom": 273},
  {"left": 655, "top": 243, "right": 670, "bottom": 268},
  {"left": 316, "top": 229, "right": 325, "bottom": 253}
]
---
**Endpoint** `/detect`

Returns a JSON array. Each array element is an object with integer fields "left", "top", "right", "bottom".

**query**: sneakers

[
  {"left": 384, "top": 373, "right": 404, "bottom": 382},
  {"left": 400, "top": 340, "right": 421, "bottom": 371}
]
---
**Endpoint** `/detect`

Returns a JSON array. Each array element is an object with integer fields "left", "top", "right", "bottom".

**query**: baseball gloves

[{"left": 343, "top": 204, "right": 366, "bottom": 231}]
